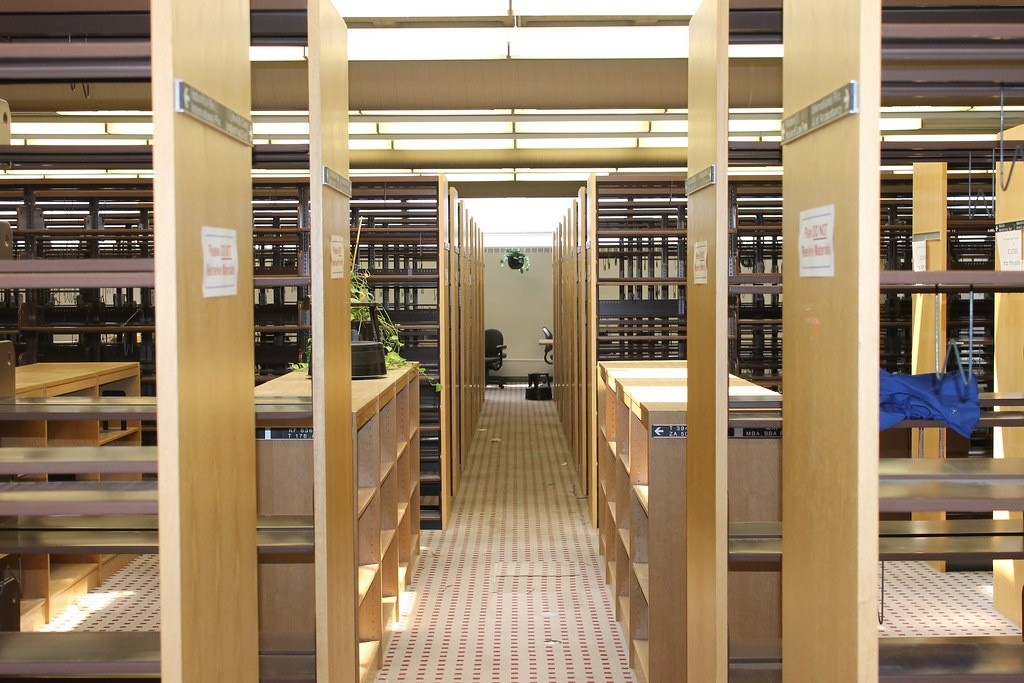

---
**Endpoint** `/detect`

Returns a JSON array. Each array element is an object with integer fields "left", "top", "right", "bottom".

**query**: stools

[{"left": 528, "top": 372, "right": 551, "bottom": 387}]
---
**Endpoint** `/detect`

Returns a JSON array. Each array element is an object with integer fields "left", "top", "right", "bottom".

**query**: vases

[{"left": 507, "top": 256, "right": 525, "bottom": 269}]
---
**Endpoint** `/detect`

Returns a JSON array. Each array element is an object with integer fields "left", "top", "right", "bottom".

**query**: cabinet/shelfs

[
  {"left": 0, "top": 0, "right": 485, "bottom": 683},
  {"left": 554, "top": 0, "right": 1024, "bottom": 683}
]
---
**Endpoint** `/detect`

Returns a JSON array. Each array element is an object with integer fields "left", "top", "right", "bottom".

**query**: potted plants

[{"left": 287, "top": 250, "right": 446, "bottom": 391}]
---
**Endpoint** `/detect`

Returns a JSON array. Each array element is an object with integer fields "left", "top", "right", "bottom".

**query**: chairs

[
  {"left": 543, "top": 327, "right": 554, "bottom": 364},
  {"left": 633, "top": 332, "right": 659, "bottom": 353},
  {"left": 484, "top": 328, "right": 507, "bottom": 389},
  {"left": 599, "top": 332, "right": 616, "bottom": 355}
]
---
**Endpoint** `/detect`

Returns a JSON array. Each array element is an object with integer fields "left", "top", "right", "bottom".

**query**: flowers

[{"left": 500, "top": 247, "right": 531, "bottom": 274}]
